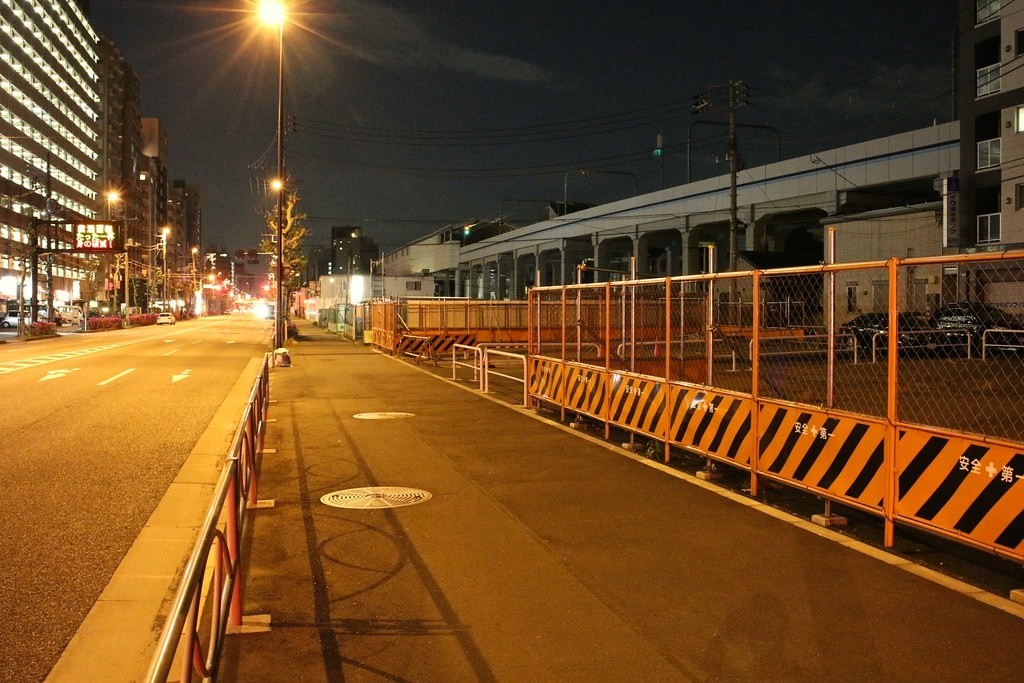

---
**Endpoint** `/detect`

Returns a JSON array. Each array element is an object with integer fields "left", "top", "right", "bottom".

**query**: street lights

[
  {"left": 191, "top": 248, "right": 198, "bottom": 291},
  {"left": 106, "top": 190, "right": 124, "bottom": 316},
  {"left": 161, "top": 226, "right": 171, "bottom": 313},
  {"left": 248, "top": 0, "right": 293, "bottom": 369},
  {"left": 200, "top": 275, "right": 214, "bottom": 295}
]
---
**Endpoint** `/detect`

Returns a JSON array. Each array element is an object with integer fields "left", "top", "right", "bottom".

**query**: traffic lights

[{"left": 465, "top": 227, "right": 470, "bottom": 235}]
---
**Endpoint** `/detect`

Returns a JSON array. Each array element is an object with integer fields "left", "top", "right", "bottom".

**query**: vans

[{"left": 8, "top": 304, "right": 67, "bottom": 326}]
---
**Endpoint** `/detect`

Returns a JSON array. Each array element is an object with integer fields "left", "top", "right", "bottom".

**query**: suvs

[{"left": 928, "top": 301, "right": 1024, "bottom": 350}]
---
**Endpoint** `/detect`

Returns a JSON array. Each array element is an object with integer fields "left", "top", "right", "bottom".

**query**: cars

[
  {"left": 56, "top": 306, "right": 84, "bottom": 323},
  {"left": 1, "top": 311, "right": 48, "bottom": 329},
  {"left": 156, "top": 313, "right": 176, "bottom": 325},
  {"left": 839, "top": 311, "right": 952, "bottom": 358}
]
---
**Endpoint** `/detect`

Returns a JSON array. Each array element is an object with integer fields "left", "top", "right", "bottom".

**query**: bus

[{"left": 65, "top": 299, "right": 90, "bottom": 318}]
